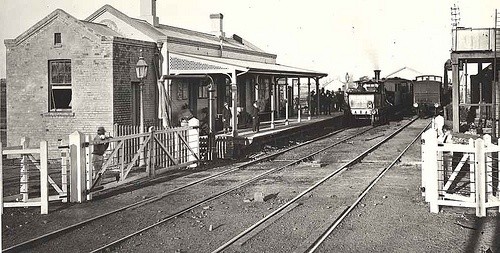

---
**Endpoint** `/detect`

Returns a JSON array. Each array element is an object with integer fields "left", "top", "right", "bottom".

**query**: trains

[
  {"left": 346, "top": 70, "right": 413, "bottom": 125},
  {"left": 413, "top": 74, "right": 452, "bottom": 117}
]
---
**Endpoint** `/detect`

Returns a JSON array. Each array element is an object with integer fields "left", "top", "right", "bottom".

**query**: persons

[
  {"left": 91, "top": 125, "right": 112, "bottom": 176},
  {"left": 221, "top": 99, "right": 233, "bottom": 134},
  {"left": 250, "top": 98, "right": 261, "bottom": 134},
  {"left": 307, "top": 86, "right": 344, "bottom": 115}
]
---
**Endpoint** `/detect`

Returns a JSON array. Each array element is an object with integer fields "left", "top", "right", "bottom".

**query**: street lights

[{"left": 135, "top": 49, "right": 148, "bottom": 167}]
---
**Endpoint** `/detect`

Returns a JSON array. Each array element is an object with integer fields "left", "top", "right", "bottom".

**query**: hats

[
  {"left": 331, "top": 90, "right": 335, "bottom": 93},
  {"left": 311, "top": 91, "right": 315, "bottom": 93},
  {"left": 98, "top": 127, "right": 106, "bottom": 132},
  {"left": 224, "top": 102, "right": 229, "bottom": 106}
]
